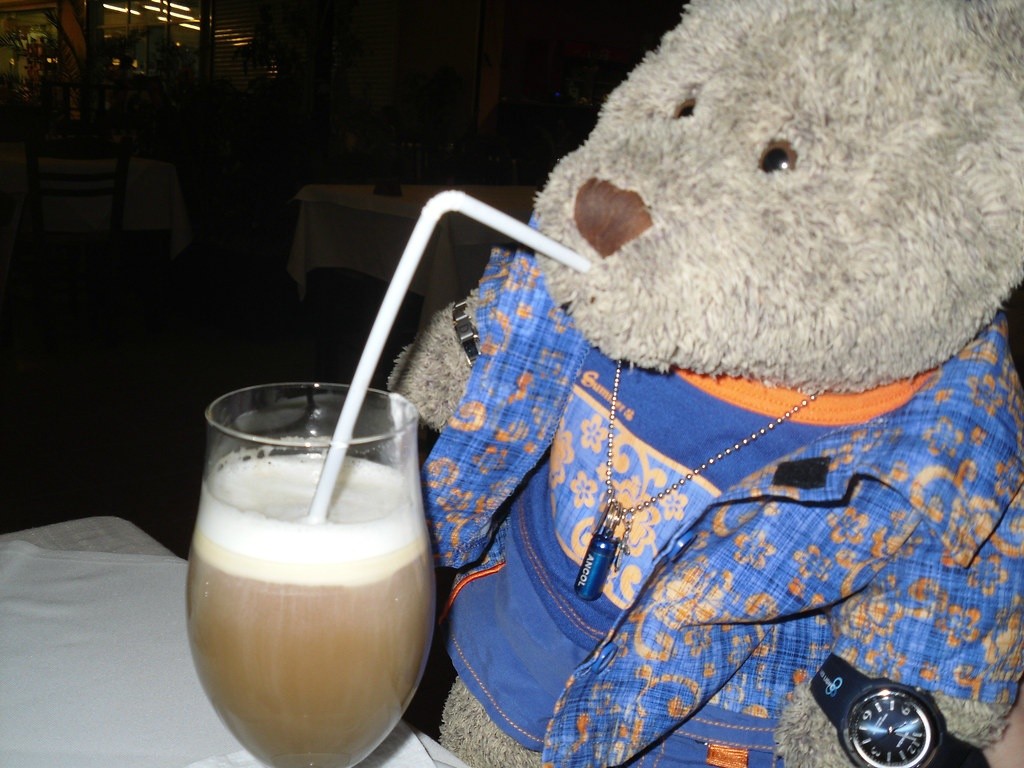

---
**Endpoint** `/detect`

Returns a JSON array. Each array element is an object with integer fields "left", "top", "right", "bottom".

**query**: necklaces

[{"left": 576, "top": 361, "right": 816, "bottom": 600}]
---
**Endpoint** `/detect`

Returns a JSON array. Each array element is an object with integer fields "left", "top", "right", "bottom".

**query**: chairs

[
  {"left": 0, "top": 191, "right": 25, "bottom": 353},
  {"left": 26, "top": 152, "right": 128, "bottom": 338}
]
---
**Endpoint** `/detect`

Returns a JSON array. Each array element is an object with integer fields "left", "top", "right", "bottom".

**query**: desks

[
  {"left": 1, "top": 515, "right": 471, "bottom": 768},
  {"left": 286, "top": 185, "right": 543, "bottom": 384},
  {"left": 1, "top": 139, "right": 194, "bottom": 337}
]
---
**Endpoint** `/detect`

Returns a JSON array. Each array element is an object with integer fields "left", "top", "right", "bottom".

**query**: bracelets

[{"left": 453, "top": 298, "right": 482, "bottom": 368}]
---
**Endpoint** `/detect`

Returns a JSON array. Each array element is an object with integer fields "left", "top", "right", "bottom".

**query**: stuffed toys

[{"left": 389, "top": 0, "right": 1024, "bottom": 768}]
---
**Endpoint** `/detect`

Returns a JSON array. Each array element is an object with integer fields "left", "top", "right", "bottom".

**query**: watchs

[{"left": 809, "top": 653, "right": 991, "bottom": 768}]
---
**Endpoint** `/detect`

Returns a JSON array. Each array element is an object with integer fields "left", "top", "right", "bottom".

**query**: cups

[{"left": 186, "top": 381, "right": 433, "bottom": 768}]
